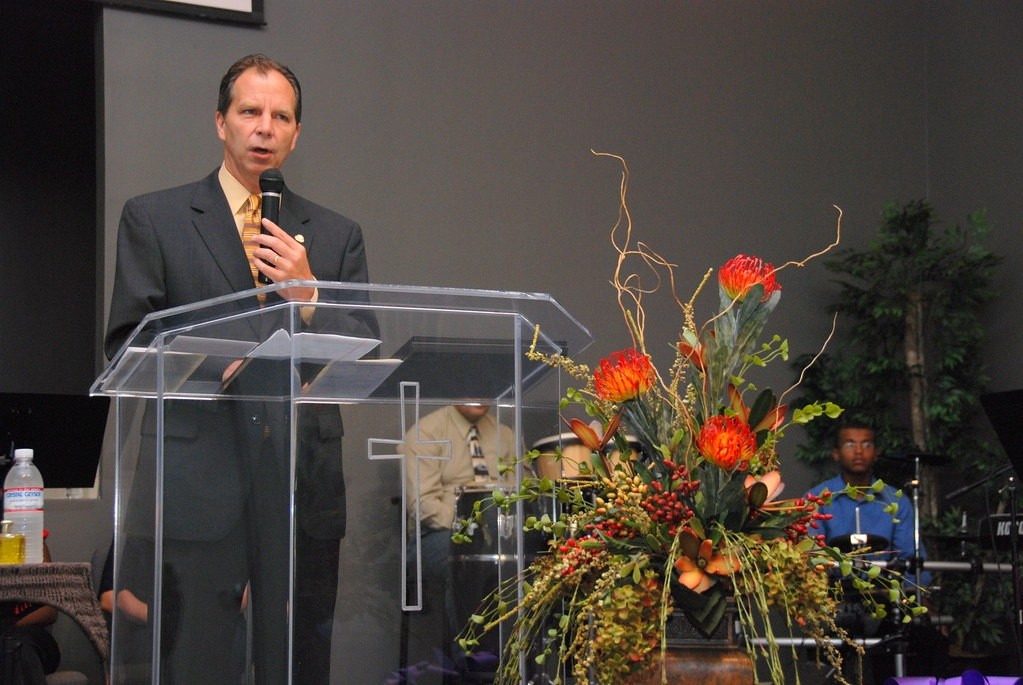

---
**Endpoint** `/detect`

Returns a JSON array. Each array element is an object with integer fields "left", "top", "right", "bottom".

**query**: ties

[
  {"left": 467, "top": 427, "right": 492, "bottom": 482},
  {"left": 241, "top": 194, "right": 266, "bottom": 316}
]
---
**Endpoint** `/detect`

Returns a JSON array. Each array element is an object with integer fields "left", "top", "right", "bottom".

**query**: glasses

[{"left": 839, "top": 441, "right": 871, "bottom": 450}]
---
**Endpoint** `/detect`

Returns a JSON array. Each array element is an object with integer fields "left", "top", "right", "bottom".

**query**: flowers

[{"left": 450, "top": 150, "right": 933, "bottom": 685}]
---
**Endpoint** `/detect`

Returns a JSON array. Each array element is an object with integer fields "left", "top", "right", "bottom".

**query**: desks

[{"left": 0, "top": 561, "right": 127, "bottom": 685}]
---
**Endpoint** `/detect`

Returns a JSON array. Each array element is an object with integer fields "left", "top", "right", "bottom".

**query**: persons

[
  {"left": 801, "top": 422, "right": 932, "bottom": 685},
  {"left": 396, "top": 403, "right": 531, "bottom": 577},
  {"left": 0, "top": 524, "right": 60, "bottom": 685},
  {"left": 99, "top": 534, "right": 247, "bottom": 674},
  {"left": 114, "top": 535, "right": 157, "bottom": 665},
  {"left": 106, "top": 55, "right": 381, "bottom": 685}
]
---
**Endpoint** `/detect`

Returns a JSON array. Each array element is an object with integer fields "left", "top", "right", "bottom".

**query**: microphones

[{"left": 259, "top": 168, "right": 285, "bottom": 282}]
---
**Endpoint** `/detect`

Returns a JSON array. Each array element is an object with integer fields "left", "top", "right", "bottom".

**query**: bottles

[
  {"left": 0, "top": 520, "right": 26, "bottom": 564},
  {"left": 3, "top": 449, "right": 45, "bottom": 564}
]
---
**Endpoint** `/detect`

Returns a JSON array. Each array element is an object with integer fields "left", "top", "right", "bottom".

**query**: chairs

[
  {"left": 45, "top": 544, "right": 110, "bottom": 685},
  {"left": 390, "top": 496, "right": 450, "bottom": 685}
]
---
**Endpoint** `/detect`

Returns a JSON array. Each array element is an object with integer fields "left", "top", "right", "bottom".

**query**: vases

[{"left": 613, "top": 596, "right": 754, "bottom": 685}]
